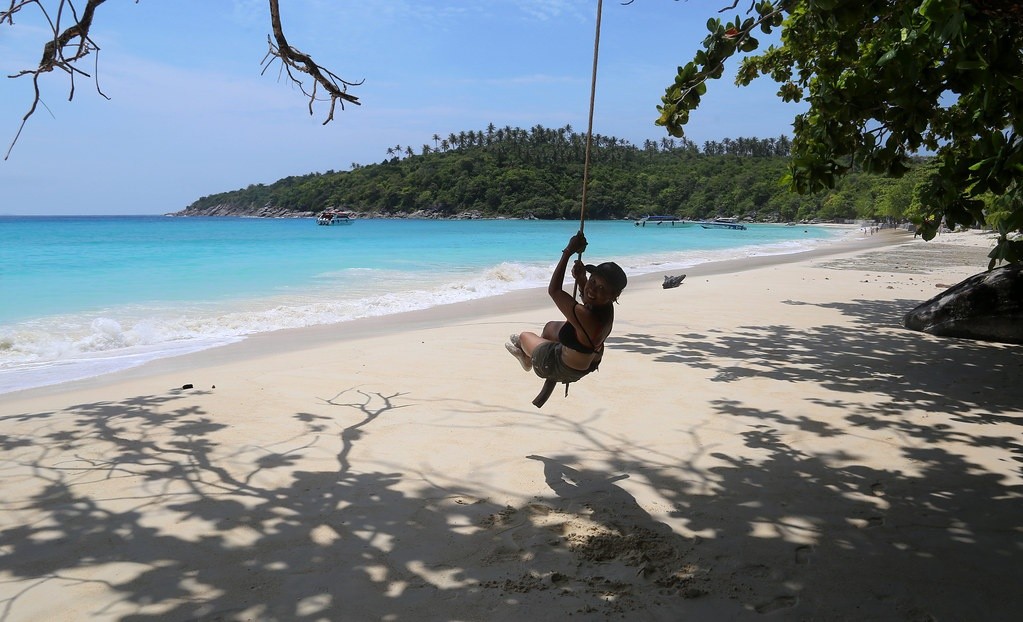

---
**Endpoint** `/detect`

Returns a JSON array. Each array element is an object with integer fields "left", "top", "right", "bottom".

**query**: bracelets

[{"left": 562, "top": 248, "right": 572, "bottom": 257}]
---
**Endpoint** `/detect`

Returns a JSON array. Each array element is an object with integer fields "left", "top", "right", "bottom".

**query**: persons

[{"left": 504, "top": 232, "right": 627, "bottom": 384}]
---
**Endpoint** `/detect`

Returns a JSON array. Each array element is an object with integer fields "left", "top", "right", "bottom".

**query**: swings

[{"left": 531, "top": 1, "right": 603, "bottom": 408}]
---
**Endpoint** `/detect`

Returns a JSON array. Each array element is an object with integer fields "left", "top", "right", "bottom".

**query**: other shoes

[
  {"left": 505, "top": 342, "right": 532, "bottom": 372},
  {"left": 510, "top": 334, "right": 522, "bottom": 349}
]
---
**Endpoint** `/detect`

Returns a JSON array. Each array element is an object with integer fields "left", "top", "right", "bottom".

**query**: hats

[{"left": 584, "top": 262, "right": 627, "bottom": 292}]
